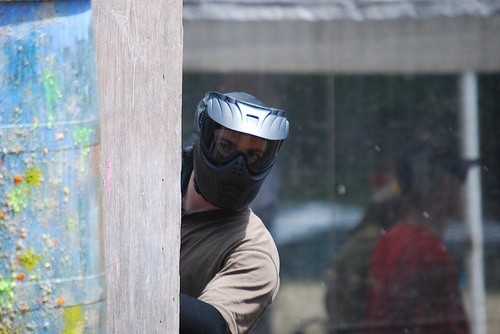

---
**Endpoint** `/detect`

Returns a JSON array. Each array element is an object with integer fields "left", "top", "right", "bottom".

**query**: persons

[
  {"left": 324, "top": 161, "right": 399, "bottom": 333},
  {"left": 180, "top": 90, "right": 290, "bottom": 334},
  {"left": 367, "top": 150, "right": 473, "bottom": 334}
]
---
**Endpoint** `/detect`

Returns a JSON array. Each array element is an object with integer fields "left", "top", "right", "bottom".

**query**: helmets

[{"left": 192, "top": 89, "right": 291, "bottom": 213}]
